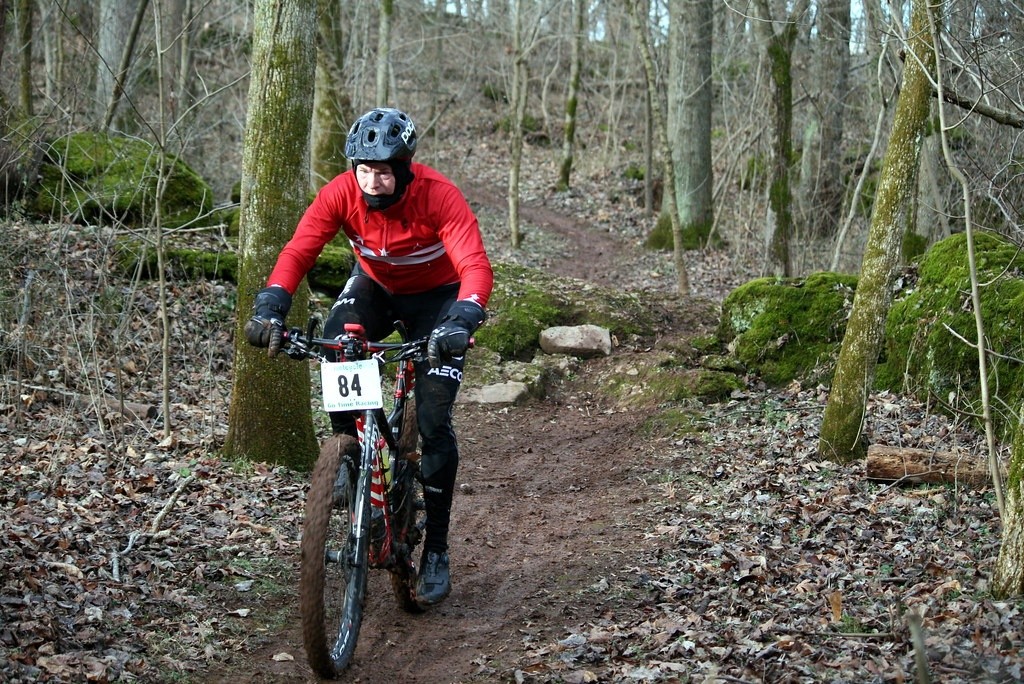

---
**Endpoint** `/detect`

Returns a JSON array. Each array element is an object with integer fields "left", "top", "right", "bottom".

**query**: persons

[{"left": 243, "top": 108, "right": 496, "bottom": 606}]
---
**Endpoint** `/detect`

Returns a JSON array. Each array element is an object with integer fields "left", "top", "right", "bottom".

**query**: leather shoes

[
  {"left": 330, "top": 448, "right": 365, "bottom": 508},
  {"left": 416, "top": 550, "right": 450, "bottom": 605}
]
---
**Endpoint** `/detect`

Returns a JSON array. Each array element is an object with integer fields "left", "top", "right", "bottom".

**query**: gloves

[
  {"left": 429, "top": 301, "right": 487, "bottom": 367},
  {"left": 244, "top": 285, "right": 292, "bottom": 358}
]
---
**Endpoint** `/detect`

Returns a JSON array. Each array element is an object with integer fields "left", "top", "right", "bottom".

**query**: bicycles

[{"left": 239, "top": 315, "right": 476, "bottom": 680}]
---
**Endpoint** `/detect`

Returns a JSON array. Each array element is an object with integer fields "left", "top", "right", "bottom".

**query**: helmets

[{"left": 345, "top": 108, "right": 418, "bottom": 164}]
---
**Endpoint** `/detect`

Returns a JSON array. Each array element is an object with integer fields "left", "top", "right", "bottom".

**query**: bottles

[{"left": 376, "top": 437, "right": 391, "bottom": 487}]
